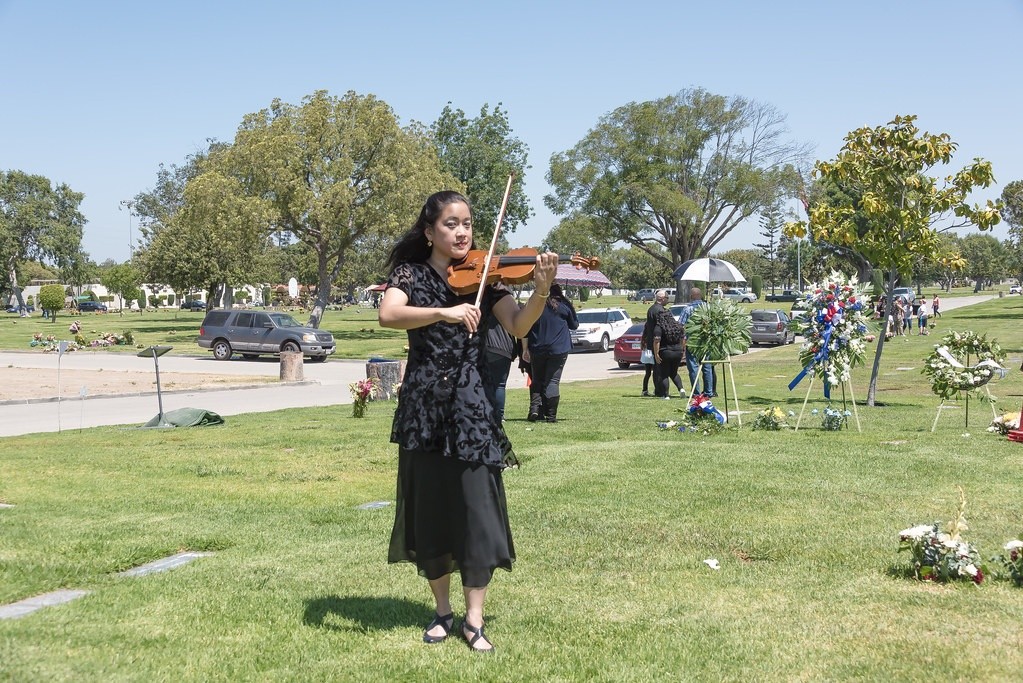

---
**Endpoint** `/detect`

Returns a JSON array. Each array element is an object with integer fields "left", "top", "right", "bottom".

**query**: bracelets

[{"left": 534, "top": 290, "right": 550, "bottom": 298}]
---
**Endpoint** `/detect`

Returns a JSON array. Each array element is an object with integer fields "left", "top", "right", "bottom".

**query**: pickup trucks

[{"left": 764, "top": 290, "right": 802, "bottom": 304}]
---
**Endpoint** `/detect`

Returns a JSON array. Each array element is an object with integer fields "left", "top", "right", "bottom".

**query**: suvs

[
  {"left": 886, "top": 287, "right": 917, "bottom": 305},
  {"left": 627, "top": 288, "right": 656, "bottom": 301},
  {"left": 709, "top": 287, "right": 757, "bottom": 303},
  {"left": 333, "top": 295, "right": 359, "bottom": 306},
  {"left": 568, "top": 307, "right": 634, "bottom": 354},
  {"left": 746, "top": 308, "right": 796, "bottom": 345},
  {"left": 198, "top": 308, "right": 337, "bottom": 363},
  {"left": 76, "top": 301, "right": 108, "bottom": 312}
]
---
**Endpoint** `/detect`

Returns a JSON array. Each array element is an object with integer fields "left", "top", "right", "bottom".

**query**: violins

[{"left": 446, "top": 249, "right": 601, "bottom": 295}]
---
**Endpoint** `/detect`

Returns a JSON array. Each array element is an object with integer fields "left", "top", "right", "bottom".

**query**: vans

[{"left": 653, "top": 288, "right": 678, "bottom": 303}]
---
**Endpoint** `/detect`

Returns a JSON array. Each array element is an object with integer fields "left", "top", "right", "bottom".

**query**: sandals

[
  {"left": 423, "top": 612, "right": 455, "bottom": 643},
  {"left": 459, "top": 615, "right": 495, "bottom": 656}
]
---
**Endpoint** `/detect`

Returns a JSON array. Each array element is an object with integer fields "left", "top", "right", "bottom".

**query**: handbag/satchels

[{"left": 640, "top": 349, "right": 655, "bottom": 365}]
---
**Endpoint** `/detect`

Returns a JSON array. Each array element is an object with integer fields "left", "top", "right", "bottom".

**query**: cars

[
  {"left": 6, "top": 304, "right": 35, "bottom": 313},
  {"left": 248, "top": 299, "right": 279, "bottom": 307},
  {"left": 951, "top": 275, "right": 1003, "bottom": 288},
  {"left": 790, "top": 298, "right": 818, "bottom": 321},
  {"left": 667, "top": 304, "right": 693, "bottom": 339},
  {"left": 1009, "top": 285, "right": 1022, "bottom": 294},
  {"left": 613, "top": 322, "right": 654, "bottom": 368},
  {"left": 180, "top": 301, "right": 207, "bottom": 309}
]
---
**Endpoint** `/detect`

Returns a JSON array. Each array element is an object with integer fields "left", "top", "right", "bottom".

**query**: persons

[
  {"left": 932, "top": 293, "right": 942, "bottom": 318},
  {"left": 641, "top": 292, "right": 686, "bottom": 399},
  {"left": 378, "top": 192, "right": 558, "bottom": 655},
  {"left": 379, "top": 295, "right": 383, "bottom": 306},
  {"left": 517, "top": 284, "right": 580, "bottom": 423},
  {"left": 678, "top": 287, "right": 714, "bottom": 398},
  {"left": 69, "top": 320, "right": 81, "bottom": 334},
  {"left": 373, "top": 294, "right": 378, "bottom": 309},
  {"left": 893, "top": 295, "right": 928, "bottom": 337}
]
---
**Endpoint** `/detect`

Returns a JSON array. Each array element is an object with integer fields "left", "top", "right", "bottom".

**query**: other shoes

[
  {"left": 680, "top": 388, "right": 686, "bottom": 398},
  {"left": 660, "top": 395, "right": 670, "bottom": 400},
  {"left": 641, "top": 390, "right": 650, "bottom": 396}
]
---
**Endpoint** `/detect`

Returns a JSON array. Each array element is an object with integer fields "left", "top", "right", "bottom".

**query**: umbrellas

[
  {"left": 551, "top": 263, "right": 610, "bottom": 299},
  {"left": 371, "top": 282, "right": 387, "bottom": 291},
  {"left": 670, "top": 253, "right": 747, "bottom": 303}
]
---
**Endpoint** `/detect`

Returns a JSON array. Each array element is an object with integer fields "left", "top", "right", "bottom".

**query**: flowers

[
  {"left": 921, "top": 330, "right": 1010, "bottom": 405},
  {"left": 789, "top": 266, "right": 879, "bottom": 391}
]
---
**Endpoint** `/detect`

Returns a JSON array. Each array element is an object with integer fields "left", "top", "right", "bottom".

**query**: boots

[
  {"left": 528, "top": 401, "right": 540, "bottom": 420},
  {"left": 543, "top": 393, "right": 560, "bottom": 423}
]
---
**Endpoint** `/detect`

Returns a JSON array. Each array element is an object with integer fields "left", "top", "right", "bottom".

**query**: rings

[{"left": 544, "top": 262, "right": 548, "bottom": 266}]
